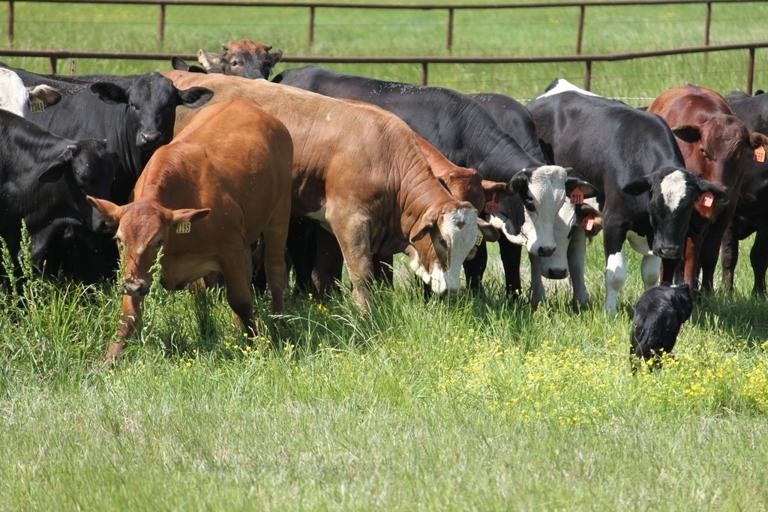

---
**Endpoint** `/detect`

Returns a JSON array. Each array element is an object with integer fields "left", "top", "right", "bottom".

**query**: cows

[
  {"left": 0, "top": 64, "right": 214, "bottom": 205},
  {"left": 85, "top": 95, "right": 295, "bottom": 365},
  {"left": 0, "top": 64, "right": 63, "bottom": 118},
  {"left": 717, "top": 88, "right": 767, "bottom": 300},
  {"left": 194, "top": 36, "right": 284, "bottom": 82},
  {"left": 456, "top": 91, "right": 604, "bottom": 311},
  {"left": 0, "top": 106, "right": 120, "bottom": 325},
  {"left": 644, "top": 82, "right": 768, "bottom": 303},
  {"left": 522, "top": 77, "right": 733, "bottom": 322},
  {"left": 266, "top": 64, "right": 602, "bottom": 309},
  {"left": 153, "top": 68, "right": 502, "bottom": 322}
]
